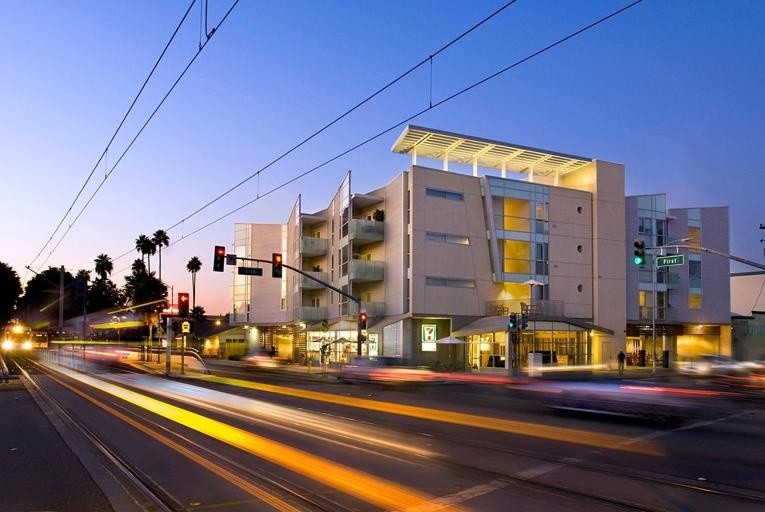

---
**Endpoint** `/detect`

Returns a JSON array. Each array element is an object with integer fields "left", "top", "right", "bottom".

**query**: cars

[
  {"left": 30, "top": 330, "right": 49, "bottom": 350},
  {"left": 680, "top": 353, "right": 749, "bottom": 375}
]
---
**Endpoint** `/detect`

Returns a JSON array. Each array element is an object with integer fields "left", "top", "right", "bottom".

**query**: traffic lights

[
  {"left": 271, "top": 252, "right": 283, "bottom": 277},
  {"left": 212, "top": 245, "right": 226, "bottom": 272},
  {"left": 633, "top": 239, "right": 645, "bottom": 266},
  {"left": 509, "top": 310, "right": 517, "bottom": 329},
  {"left": 177, "top": 292, "right": 189, "bottom": 315},
  {"left": 521, "top": 313, "right": 529, "bottom": 331},
  {"left": 359, "top": 312, "right": 367, "bottom": 329}
]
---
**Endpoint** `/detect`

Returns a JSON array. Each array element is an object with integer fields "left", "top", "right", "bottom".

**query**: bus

[{"left": 1, "top": 321, "right": 32, "bottom": 353}]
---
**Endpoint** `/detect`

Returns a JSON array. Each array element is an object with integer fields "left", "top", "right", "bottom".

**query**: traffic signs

[
  {"left": 237, "top": 266, "right": 262, "bottom": 276},
  {"left": 656, "top": 254, "right": 684, "bottom": 267}
]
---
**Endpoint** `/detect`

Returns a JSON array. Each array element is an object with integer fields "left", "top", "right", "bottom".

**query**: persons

[{"left": 616, "top": 350, "right": 625, "bottom": 375}]
